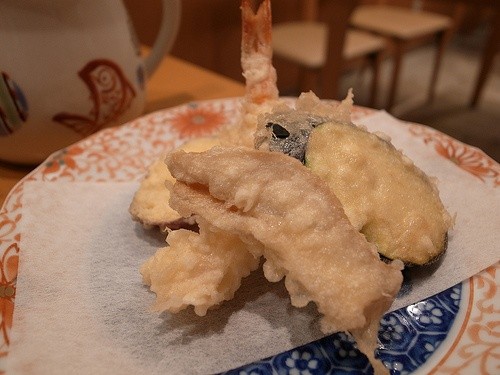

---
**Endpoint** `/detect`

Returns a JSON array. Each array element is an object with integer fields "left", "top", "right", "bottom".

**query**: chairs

[
  {"left": 249, "top": 0, "right": 388, "bottom": 108},
  {"left": 349, "top": 0, "right": 453, "bottom": 112}
]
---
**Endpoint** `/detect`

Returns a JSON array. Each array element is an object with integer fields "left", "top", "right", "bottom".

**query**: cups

[{"left": 0, "top": 0, "right": 180, "bottom": 166}]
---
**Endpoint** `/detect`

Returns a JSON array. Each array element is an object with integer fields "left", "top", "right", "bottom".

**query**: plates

[{"left": 0, "top": 96, "right": 499, "bottom": 374}]
